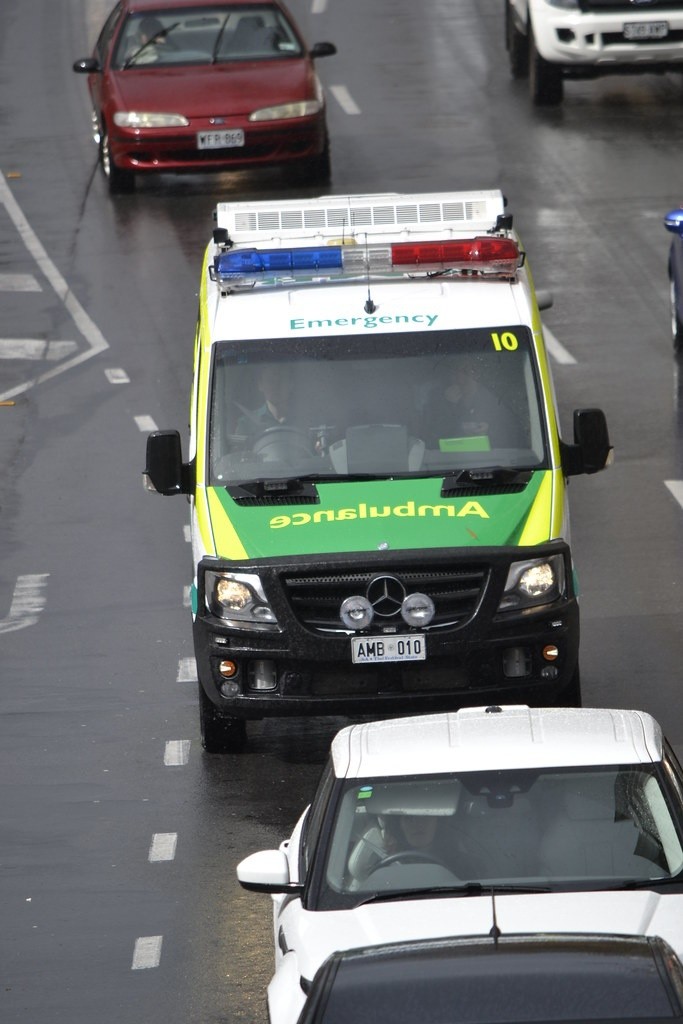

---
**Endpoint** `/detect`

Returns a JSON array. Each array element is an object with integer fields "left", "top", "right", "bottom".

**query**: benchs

[{"left": 123, "top": 26, "right": 274, "bottom": 58}]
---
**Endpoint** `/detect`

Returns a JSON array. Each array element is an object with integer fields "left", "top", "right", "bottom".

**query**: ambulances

[{"left": 141, "top": 186, "right": 613, "bottom": 756}]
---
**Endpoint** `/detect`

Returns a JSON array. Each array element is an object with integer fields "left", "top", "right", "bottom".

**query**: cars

[
  {"left": 72, "top": 1, "right": 339, "bottom": 196},
  {"left": 503, "top": 1, "right": 683, "bottom": 108},
  {"left": 297, "top": 931, "right": 683, "bottom": 1024}
]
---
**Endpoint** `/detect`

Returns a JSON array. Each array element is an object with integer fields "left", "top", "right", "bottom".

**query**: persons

[
  {"left": 235, "top": 354, "right": 322, "bottom": 449},
  {"left": 369, "top": 813, "right": 487, "bottom": 880},
  {"left": 429, "top": 338, "right": 532, "bottom": 451},
  {"left": 123, "top": 20, "right": 176, "bottom": 66}
]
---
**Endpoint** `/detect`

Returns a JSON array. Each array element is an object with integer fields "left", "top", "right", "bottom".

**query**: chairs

[
  {"left": 539, "top": 780, "right": 665, "bottom": 878},
  {"left": 348, "top": 818, "right": 397, "bottom": 892},
  {"left": 222, "top": 16, "right": 283, "bottom": 56},
  {"left": 450, "top": 785, "right": 542, "bottom": 879}
]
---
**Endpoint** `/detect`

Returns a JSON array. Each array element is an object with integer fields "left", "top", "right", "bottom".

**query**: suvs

[{"left": 234, "top": 704, "right": 683, "bottom": 1024}]
